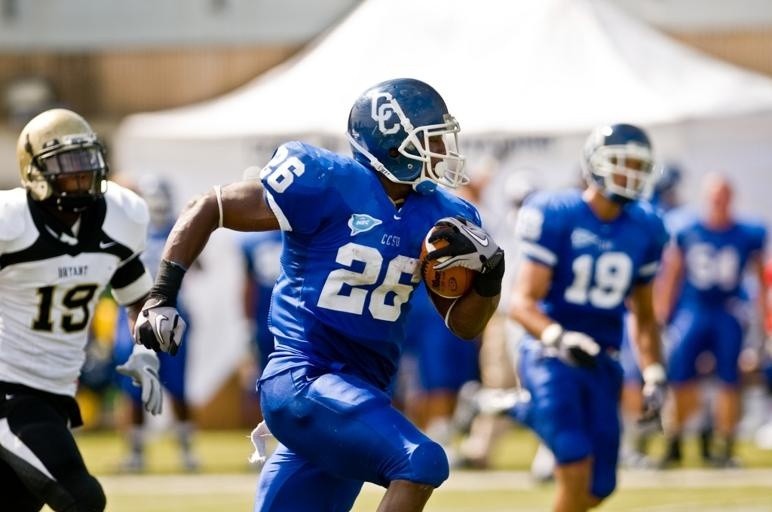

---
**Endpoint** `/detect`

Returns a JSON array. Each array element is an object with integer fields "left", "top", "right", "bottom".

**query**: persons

[
  {"left": 0, "top": 106, "right": 163, "bottom": 512},
  {"left": 130, "top": 77, "right": 506, "bottom": 512},
  {"left": 505, "top": 123, "right": 677, "bottom": 511},
  {"left": 401, "top": 171, "right": 554, "bottom": 481},
  {"left": 624, "top": 163, "right": 770, "bottom": 471},
  {"left": 71, "top": 175, "right": 202, "bottom": 477},
  {"left": 238, "top": 227, "right": 290, "bottom": 471}
]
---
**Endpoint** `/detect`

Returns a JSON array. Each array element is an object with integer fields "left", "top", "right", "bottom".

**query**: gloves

[
  {"left": 539, "top": 326, "right": 600, "bottom": 369},
  {"left": 133, "top": 260, "right": 188, "bottom": 358},
  {"left": 115, "top": 345, "right": 163, "bottom": 417},
  {"left": 428, "top": 214, "right": 506, "bottom": 298},
  {"left": 637, "top": 363, "right": 667, "bottom": 425}
]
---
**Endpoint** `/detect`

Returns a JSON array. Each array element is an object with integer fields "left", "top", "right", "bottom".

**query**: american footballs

[{"left": 421, "top": 225, "right": 475, "bottom": 299}]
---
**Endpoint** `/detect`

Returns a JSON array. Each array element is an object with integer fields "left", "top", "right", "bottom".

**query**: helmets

[
  {"left": 580, "top": 123, "right": 653, "bottom": 205},
  {"left": 14, "top": 108, "right": 111, "bottom": 212},
  {"left": 347, "top": 79, "right": 469, "bottom": 195}
]
